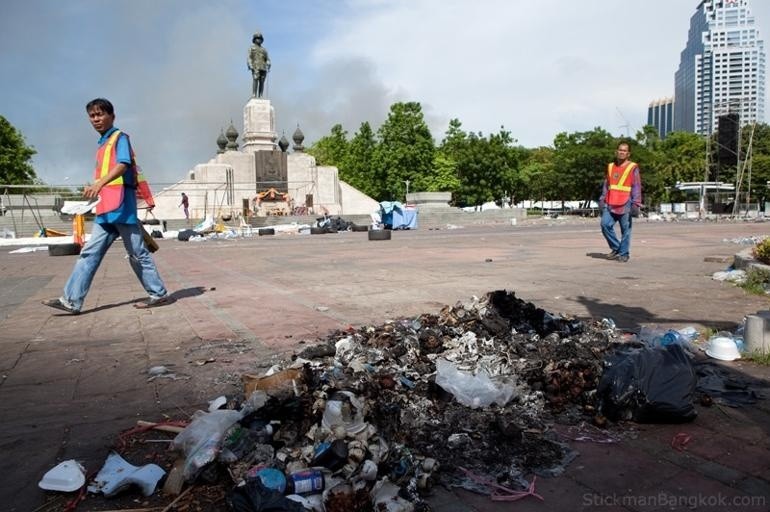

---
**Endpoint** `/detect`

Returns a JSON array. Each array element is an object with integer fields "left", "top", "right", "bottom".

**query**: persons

[
  {"left": 178, "top": 192, "right": 189, "bottom": 220},
  {"left": 38, "top": 97, "right": 169, "bottom": 314},
  {"left": 247, "top": 32, "right": 271, "bottom": 98},
  {"left": 598, "top": 141, "right": 642, "bottom": 263}
]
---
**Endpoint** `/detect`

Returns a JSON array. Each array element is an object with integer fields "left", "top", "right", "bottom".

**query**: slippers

[
  {"left": 41, "top": 298, "right": 80, "bottom": 315},
  {"left": 134, "top": 298, "right": 169, "bottom": 309}
]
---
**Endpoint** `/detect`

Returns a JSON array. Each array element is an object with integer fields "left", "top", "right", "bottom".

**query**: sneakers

[{"left": 606, "top": 249, "right": 629, "bottom": 262}]
